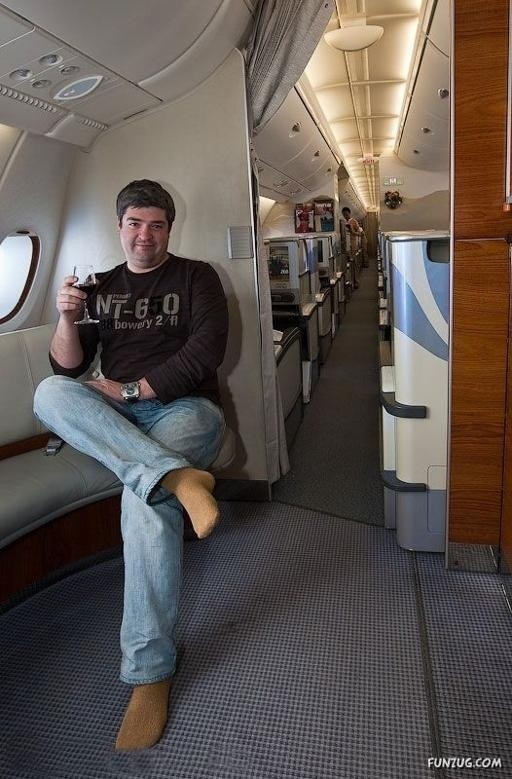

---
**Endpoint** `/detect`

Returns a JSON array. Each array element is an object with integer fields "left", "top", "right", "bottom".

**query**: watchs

[{"left": 119, "top": 380, "right": 141, "bottom": 403}]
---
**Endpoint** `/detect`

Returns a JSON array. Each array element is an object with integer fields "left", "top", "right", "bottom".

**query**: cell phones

[{"left": 45, "top": 436, "right": 63, "bottom": 454}]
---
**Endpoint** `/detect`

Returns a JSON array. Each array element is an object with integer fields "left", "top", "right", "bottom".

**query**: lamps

[{"left": 324, "top": 24, "right": 383, "bottom": 52}]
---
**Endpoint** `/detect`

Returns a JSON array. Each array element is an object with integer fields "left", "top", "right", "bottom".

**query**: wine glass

[{"left": 67, "top": 263, "right": 101, "bottom": 325}]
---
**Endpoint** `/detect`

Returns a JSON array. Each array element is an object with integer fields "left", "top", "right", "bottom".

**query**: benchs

[{"left": 1, "top": 320, "right": 121, "bottom": 621}]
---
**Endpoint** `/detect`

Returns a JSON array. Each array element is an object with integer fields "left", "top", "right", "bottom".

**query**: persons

[
  {"left": 32, "top": 181, "right": 229, "bottom": 752},
  {"left": 361, "top": 226, "right": 369, "bottom": 269},
  {"left": 342, "top": 207, "right": 360, "bottom": 252}
]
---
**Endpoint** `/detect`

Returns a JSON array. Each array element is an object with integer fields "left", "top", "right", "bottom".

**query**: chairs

[{"left": 264, "top": 230, "right": 395, "bottom": 449}]
